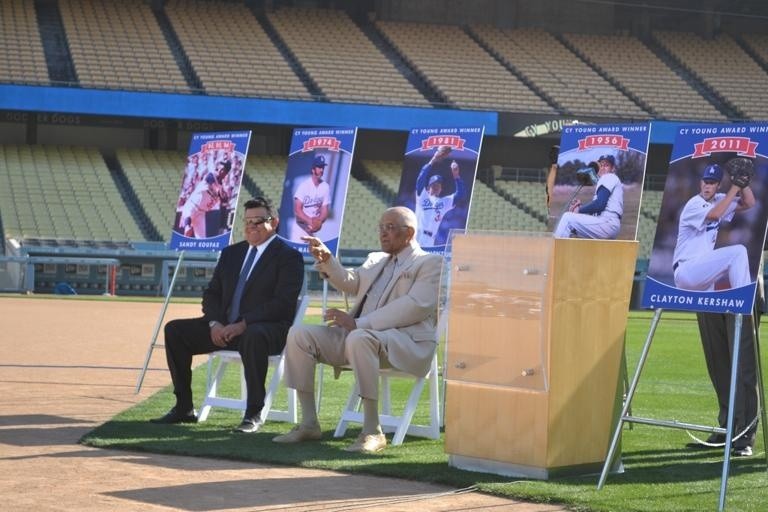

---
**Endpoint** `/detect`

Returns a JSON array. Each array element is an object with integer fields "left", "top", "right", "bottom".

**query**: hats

[
  {"left": 597, "top": 154, "right": 614, "bottom": 165},
  {"left": 310, "top": 155, "right": 327, "bottom": 169},
  {"left": 701, "top": 165, "right": 722, "bottom": 182},
  {"left": 428, "top": 174, "right": 442, "bottom": 186},
  {"left": 219, "top": 159, "right": 231, "bottom": 171}
]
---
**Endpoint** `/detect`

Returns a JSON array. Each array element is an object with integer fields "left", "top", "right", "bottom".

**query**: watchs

[{"left": 209, "top": 320, "right": 216, "bottom": 327}]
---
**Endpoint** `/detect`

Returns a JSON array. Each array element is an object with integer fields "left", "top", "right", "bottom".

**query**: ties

[
  {"left": 360, "top": 256, "right": 397, "bottom": 316},
  {"left": 226, "top": 245, "right": 257, "bottom": 324}
]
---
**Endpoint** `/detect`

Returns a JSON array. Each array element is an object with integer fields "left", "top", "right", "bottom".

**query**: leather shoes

[
  {"left": 149, "top": 407, "right": 198, "bottom": 423},
  {"left": 271, "top": 422, "right": 321, "bottom": 444},
  {"left": 339, "top": 432, "right": 387, "bottom": 454},
  {"left": 732, "top": 445, "right": 752, "bottom": 457},
  {"left": 232, "top": 417, "right": 263, "bottom": 434},
  {"left": 684, "top": 439, "right": 716, "bottom": 450}
]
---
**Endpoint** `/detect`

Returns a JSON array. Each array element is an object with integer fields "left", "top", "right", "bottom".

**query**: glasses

[
  {"left": 242, "top": 216, "right": 273, "bottom": 225},
  {"left": 377, "top": 224, "right": 408, "bottom": 231}
]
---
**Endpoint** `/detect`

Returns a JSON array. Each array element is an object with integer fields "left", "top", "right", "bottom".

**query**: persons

[
  {"left": 290, "top": 155, "right": 339, "bottom": 243},
  {"left": 150, "top": 195, "right": 305, "bottom": 434},
  {"left": 554, "top": 155, "right": 624, "bottom": 239},
  {"left": 272, "top": 206, "right": 448, "bottom": 452},
  {"left": 176, "top": 150, "right": 245, "bottom": 238},
  {"left": 673, "top": 158, "right": 756, "bottom": 291},
  {"left": 695, "top": 272, "right": 765, "bottom": 456},
  {"left": 415, "top": 146, "right": 465, "bottom": 246}
]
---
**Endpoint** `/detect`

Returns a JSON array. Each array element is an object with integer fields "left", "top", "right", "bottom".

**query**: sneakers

[{"left": 183, "top": 224, "right": 193, "bottom": 238}]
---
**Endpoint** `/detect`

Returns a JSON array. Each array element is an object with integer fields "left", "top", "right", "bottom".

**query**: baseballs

[{"left": 451, "top": 162, "right": 457, "bottom": 169}]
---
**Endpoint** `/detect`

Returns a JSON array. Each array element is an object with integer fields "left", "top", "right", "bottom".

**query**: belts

[
  {"left": 423, "top": 230, "right": 432, "bottom": 236},
  {"left": 672, "top": 262, "right": 677, "bottom": 271}
]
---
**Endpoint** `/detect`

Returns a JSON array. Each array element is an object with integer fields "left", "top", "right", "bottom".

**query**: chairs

[
  {"left": 1, "top": 143, "right": 663, "bottom": 257},
  {"left": 0, "top": 1, "right": 766, "bottom": 122},
  {"left": 333, "top": 272, "right": 450, "bottom": 446},
  {"left": 194, "top": 295, "right": 309, "bottom": 424}
]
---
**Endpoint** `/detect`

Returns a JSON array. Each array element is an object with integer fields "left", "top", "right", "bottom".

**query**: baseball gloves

[
  {"left": 724, "top": 157, "right": 757, "bottom": 189},
  {"left": 433, "top": 144, "right": 452, "bottom": 162}
]
